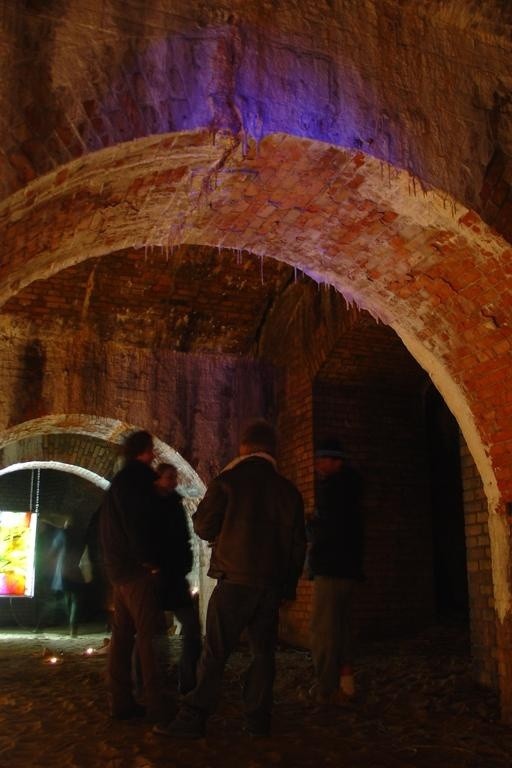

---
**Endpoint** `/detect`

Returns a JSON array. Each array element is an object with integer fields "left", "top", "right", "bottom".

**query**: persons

[
  {"left": 149, "top": 418, "right": 309, "bottom": 740},
  {"left": 51, "top": 517, "right": 88, "bottom": 639},
  {"left": 295, "top": 440, "right": 367, "bottom": 704},
  {"left": 99, "top": 428, "right": 181, "bottom": 724},
  {"left": 128, "top": 461, "right": 201, "bottom": 715}
]
